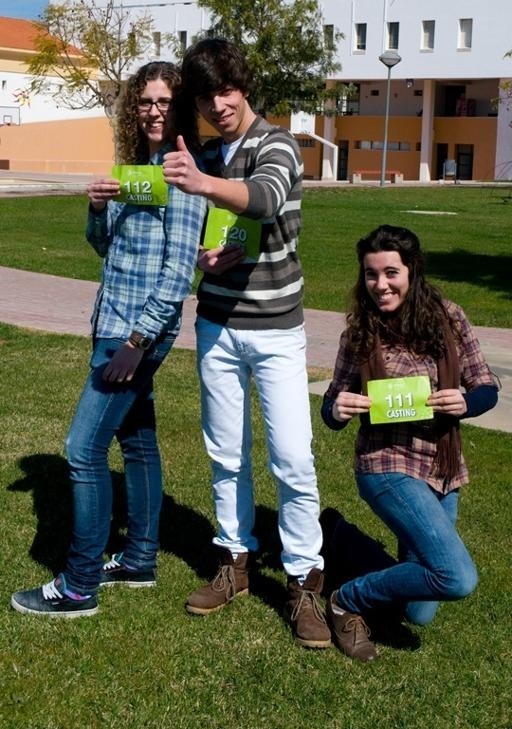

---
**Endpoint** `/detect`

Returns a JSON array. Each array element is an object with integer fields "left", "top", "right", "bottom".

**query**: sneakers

[
  {"left": 186, "top": 551, "right": 249, "bottom": 614},
  {"left": 283, "top": 568, "right": 377, "bottom": 662},
  {"left": 11, "top": 554, "right": 156, "bottom": 618}
]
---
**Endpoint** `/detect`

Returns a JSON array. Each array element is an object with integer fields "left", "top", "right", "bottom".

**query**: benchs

[{"left": 350, "top": 169, "right": 404, "bottom": 184}]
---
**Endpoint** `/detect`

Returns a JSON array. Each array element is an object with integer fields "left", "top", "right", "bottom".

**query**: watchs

[{"left": 129, "top": 330, "right": 153, "bottom": 352}]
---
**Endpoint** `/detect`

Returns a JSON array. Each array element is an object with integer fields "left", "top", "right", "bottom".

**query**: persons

[
  {"left": 163, "top": 35, "right": 332, "bottom": 651},
  {"left": 320, "top": 224, "right": 502, "bottom": 664},
  {"left": 11, "top": 59, "right": 205, "bottom": 618}
]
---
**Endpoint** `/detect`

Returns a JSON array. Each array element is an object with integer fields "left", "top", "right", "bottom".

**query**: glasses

[{"left": 139, "top": 100, "right": 174, "bottom": 112}]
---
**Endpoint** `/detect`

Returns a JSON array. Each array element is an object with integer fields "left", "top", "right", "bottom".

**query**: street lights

[{"left": 378, "top": 51, "right": 402, "bottom": 187}]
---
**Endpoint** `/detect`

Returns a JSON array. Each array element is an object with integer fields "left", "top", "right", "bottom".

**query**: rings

[{"left": 118, "top": 373, "right": 125, "bottom": 379}]
